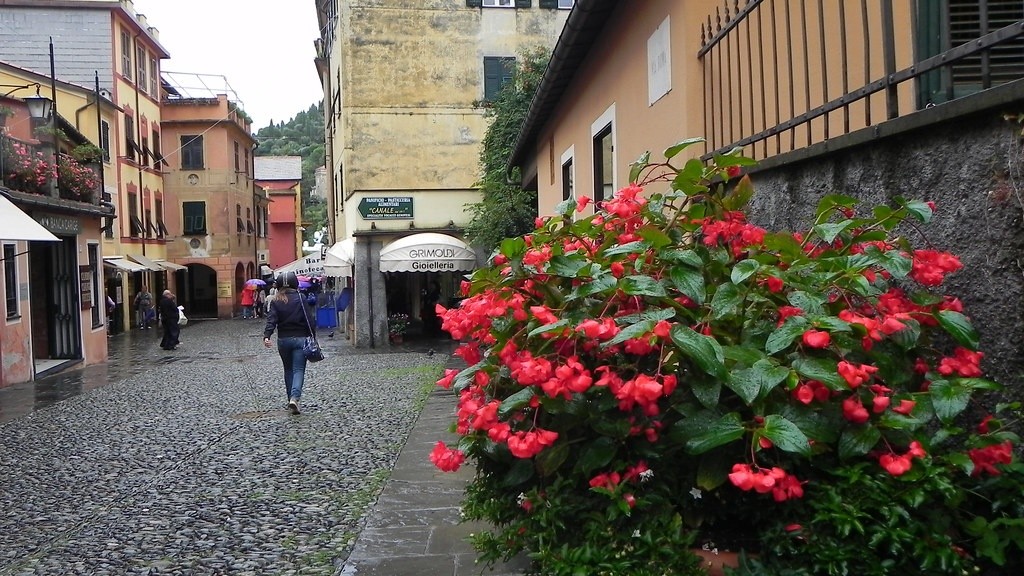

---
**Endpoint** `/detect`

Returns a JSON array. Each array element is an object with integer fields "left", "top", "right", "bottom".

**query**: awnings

[
  {"left": 127, "top": 255, "right": 165, "bottom": 271},
  {"left": 129, "top": 140, "right": 169, "bottom": 166},
  {"left": 102, "top": 256, "right": 149, "bottom": 272},
  {"left": 274, "top": 250, "right": 326, "bottom": 279},
  {"left": 0, "top": 194, "right": 62, "bottom": 241},
  {"left": 151, "top": 260, "right": 188, "bottom": 272},
  {"left": 322, "top": 239, "right": 355, "bottom": 277},
  {"left": 378, "top": 232, "right": 476, "bottom": 272},
  {"left": 131, "top": 215, "right": 168, "bottom": 235}
]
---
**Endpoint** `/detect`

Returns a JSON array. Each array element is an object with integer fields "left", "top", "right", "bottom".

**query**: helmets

[{"left": 276, "top": 272, "right": 298, "bottom": 291}]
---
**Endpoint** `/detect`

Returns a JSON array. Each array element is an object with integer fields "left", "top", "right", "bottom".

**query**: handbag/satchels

[
  {"left": 303, "top": 336, "right": 324, "bottom": 363},
  {"left": 177, "top": 309, "right": 187, "bottom": 325}
]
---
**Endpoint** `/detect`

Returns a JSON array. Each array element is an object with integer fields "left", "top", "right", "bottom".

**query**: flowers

[
  {"left": 56, "top": 153, "right": 101, "bottom": 199},
  {"left": 388, "top": 312, "right": 411, "bottom": 338},
  {"left": 2, "top": 143, "right": 58, "bottom": 193}
]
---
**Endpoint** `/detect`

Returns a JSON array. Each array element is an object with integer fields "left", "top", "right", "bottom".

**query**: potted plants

[
  {"left": 32, "top": 126, "right": 70, "bottom": 153},
  {"left": 72, "top": 143, "right": 107, "bottom": 168},
  {"left": 0, "top": 106, "right": 15, "bottom": 126}
]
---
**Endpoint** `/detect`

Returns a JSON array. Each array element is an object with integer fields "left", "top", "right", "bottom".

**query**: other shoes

[
  {"left": 244, "top": 312, "right": 268, "bottom": 319},
  {"left": 289, "top": 399, "right": 301, "bottom": 414},
  {"left": 147, "top": 326, "right": 152, "bottom": 328},
  {"left": 140, "top": 326, "right": 144, "bottom": 329}
]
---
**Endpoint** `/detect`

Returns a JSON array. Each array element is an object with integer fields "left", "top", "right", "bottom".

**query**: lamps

[{"left": 0, "top": 83, "right": 53, "bottom": 126}]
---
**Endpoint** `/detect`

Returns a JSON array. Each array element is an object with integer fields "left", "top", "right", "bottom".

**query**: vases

[{"left": 393, "top": 335, "right": 403, "bottom": 342}]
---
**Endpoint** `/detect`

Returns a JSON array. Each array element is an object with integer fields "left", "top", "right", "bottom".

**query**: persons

[
  {"left": 240, "top": 278, "right": 322, "bottom": 319},
  {"left": 133, "top": 286, "right": 153, "bottom": 329},
  {"left": 262, "top": 271, "right": 316, "bottom": 414},
  {"left": 160, "top": 290, "right": 180, "bottom": 350},
  {"left": 107, "top": 296, "right": 115, "bottom": 337}
]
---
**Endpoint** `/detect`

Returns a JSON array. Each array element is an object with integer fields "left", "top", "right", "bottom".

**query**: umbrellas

[
  {"left": 245, "top": 279, "right": 266, "bottom": 285},
  {"left": 297, "top": 282, "right": 311, "bottom": 287}
]
